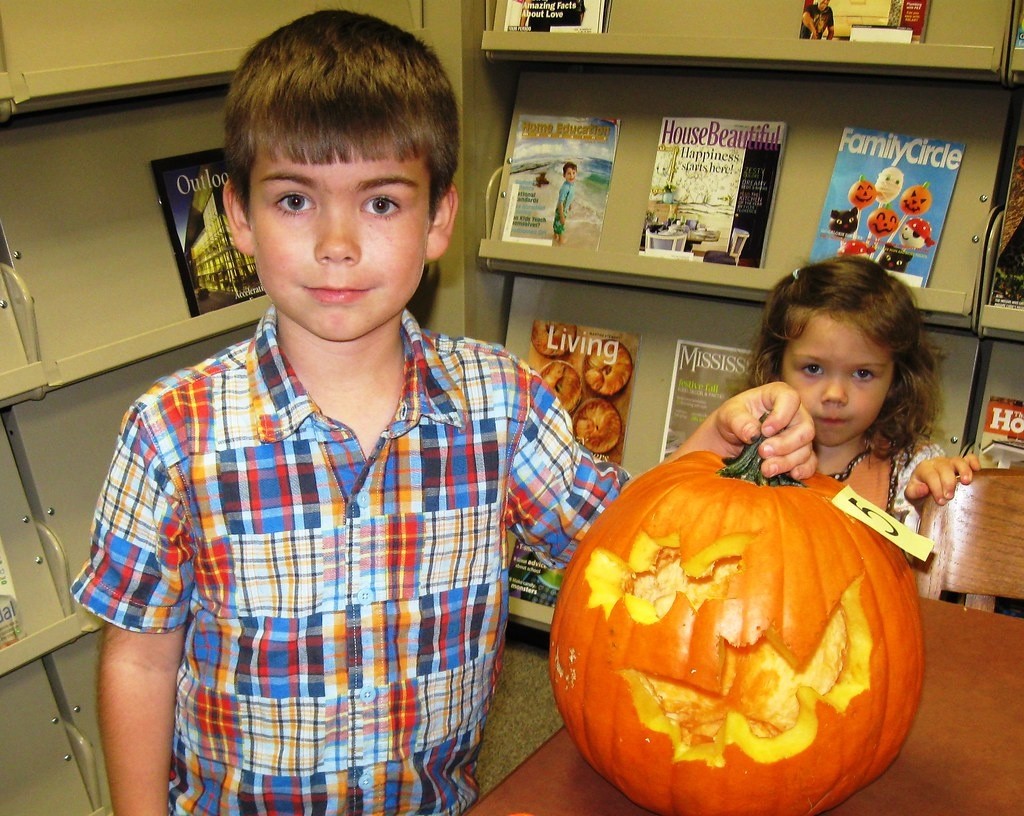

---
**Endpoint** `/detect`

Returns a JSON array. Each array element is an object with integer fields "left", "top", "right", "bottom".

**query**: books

[
  {"left": 526, "top": 318, "right": 645, "bottom": 472},
  {"left": 658, "top": 337, "right": 761, "bottom": 470},
  {"left": 977, "top": 393, "right": 1023, "bottom": 469},
  {"left": 149, "top": 147, "right": 270, "bottom": 318},
  {"left": 806, "top": 123, "right": 968, "bottom": 288},
  {"left": 987, "top": 143, "right": 1023, "bottom": 311},
  {"left": 798, "top": 0, "right": 937, "bottom": 45},
  {"left": 637, "top": 114, "right": 789, "bottom": 272},
  {"left": 501, "top": 111, "right": 623, "bottom": 253},
  {"left": 501, "top": 0, "right": 611, "bottom": 33},
  {"left": 508, "top": 527, "right": 569, "bottom": 610}
]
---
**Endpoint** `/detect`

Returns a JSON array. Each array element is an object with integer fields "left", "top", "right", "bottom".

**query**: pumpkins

[{"left": 539, "top": 421, "right": 923, "bottom": 815}]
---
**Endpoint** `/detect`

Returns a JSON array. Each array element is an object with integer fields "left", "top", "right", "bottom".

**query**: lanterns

[{"left": 546, "top": 411, "right": 928, "bottom": 816}]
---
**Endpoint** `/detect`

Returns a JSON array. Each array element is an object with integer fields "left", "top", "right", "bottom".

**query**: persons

[
  {"left": 63, "top": 6, "right": 821, "bottom": 816},
  {"left": 750, "top": 255, "right": 983, "bottom": 600},
  {"left": 549, "top": 161, "right": 578, "bottom": 249},
  {"left": 515, "top": 0, "right": 584, "bottom": 32},
  {"left": 800, "top": 0, "right": 835, "bottom": 40}
]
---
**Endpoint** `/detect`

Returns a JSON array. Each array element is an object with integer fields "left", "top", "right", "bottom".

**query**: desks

[{"left": 459, "top": 597, "right": 1024, "bottom": 815}]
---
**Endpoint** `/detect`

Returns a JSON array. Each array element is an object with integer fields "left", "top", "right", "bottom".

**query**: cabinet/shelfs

[
  {"left": 449, "top": 0, "right": 1024, "bottom": 636},
  {"left": 1, "top": 0, "right": 469, "bottom": 816}
]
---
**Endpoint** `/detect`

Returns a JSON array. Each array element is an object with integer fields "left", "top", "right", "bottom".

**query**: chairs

[{"left": 909, "top": 467, "right": 1023, "bottom": 621}]
